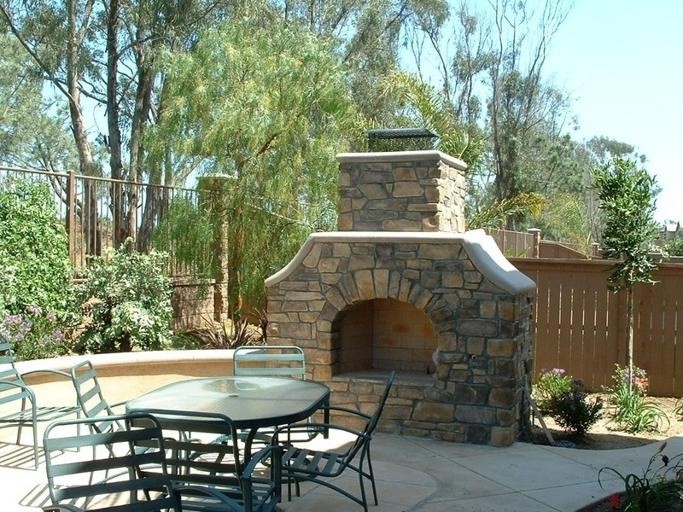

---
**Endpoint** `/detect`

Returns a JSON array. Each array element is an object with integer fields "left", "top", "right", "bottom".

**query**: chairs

[
  {"left": 261, "top": 368, "right": 394, "bottom": 510},
  {"left": 0, "top": 333, "right": 82, "bottom": 473},
  {"left": 69, "top": 360, "right": 191, "bottom": 510},
  {"left": 41, "top": 413, "right": 244, "bottom": 512},
  {"left": 125, "top": 407, "right": 283, "bottom": 511},
  {"left": 232, "top": 346, "right": 320, "bottom": 500}
]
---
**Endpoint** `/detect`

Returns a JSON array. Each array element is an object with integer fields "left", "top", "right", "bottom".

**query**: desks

[{"left": 125, "top": 376, "right": 330, "bottom": 502}]
